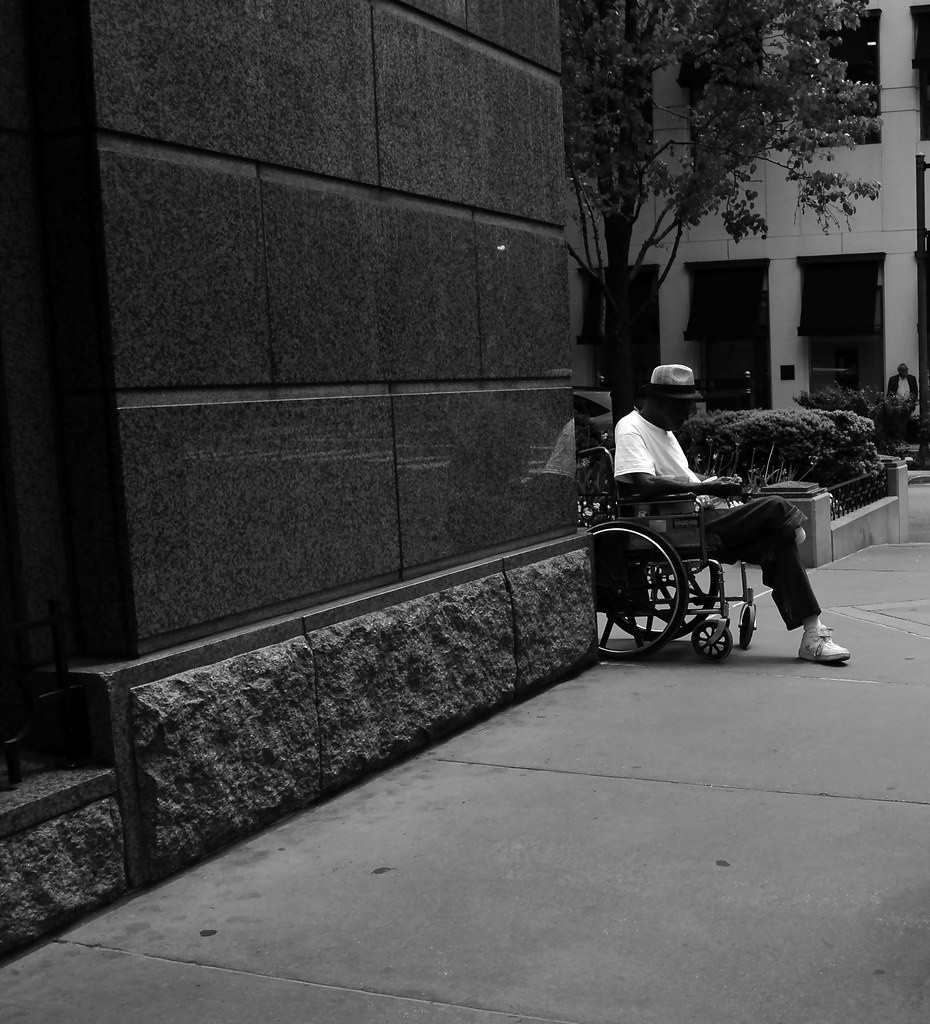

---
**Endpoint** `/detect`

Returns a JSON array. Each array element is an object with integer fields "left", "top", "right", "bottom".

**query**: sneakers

[{"left": 799, "top": 625, "right": 850, "bottom": 662}]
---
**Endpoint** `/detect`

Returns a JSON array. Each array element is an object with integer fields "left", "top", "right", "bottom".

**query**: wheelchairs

[{"left": 576, "top": 444, "right": 756, "bottom": 663}]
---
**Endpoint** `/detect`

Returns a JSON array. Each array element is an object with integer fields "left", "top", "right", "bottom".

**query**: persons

[
  {"left": 887, "top": 363, "right": 918, "bottom": 413},
  {"left": 541, "top": 418, "right": 576, "bottom": 481},
  {"left": 613, "top": 363, "right": 850, "bottom": 663}
]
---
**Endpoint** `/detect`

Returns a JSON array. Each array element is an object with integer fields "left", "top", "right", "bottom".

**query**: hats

[{"left": 640, "top": 364, "right": 701, "bottom": 400}]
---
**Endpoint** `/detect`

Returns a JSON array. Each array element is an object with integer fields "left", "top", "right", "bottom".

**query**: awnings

[
  {"left": 683, "top": 267, "right": 765, "bottom": 344},
  {"left": 575, "top": 272, "right": 653, "bottom": 345},
  {"left": 797, "top": 261, "right": 879, "bottom": 336}
]
